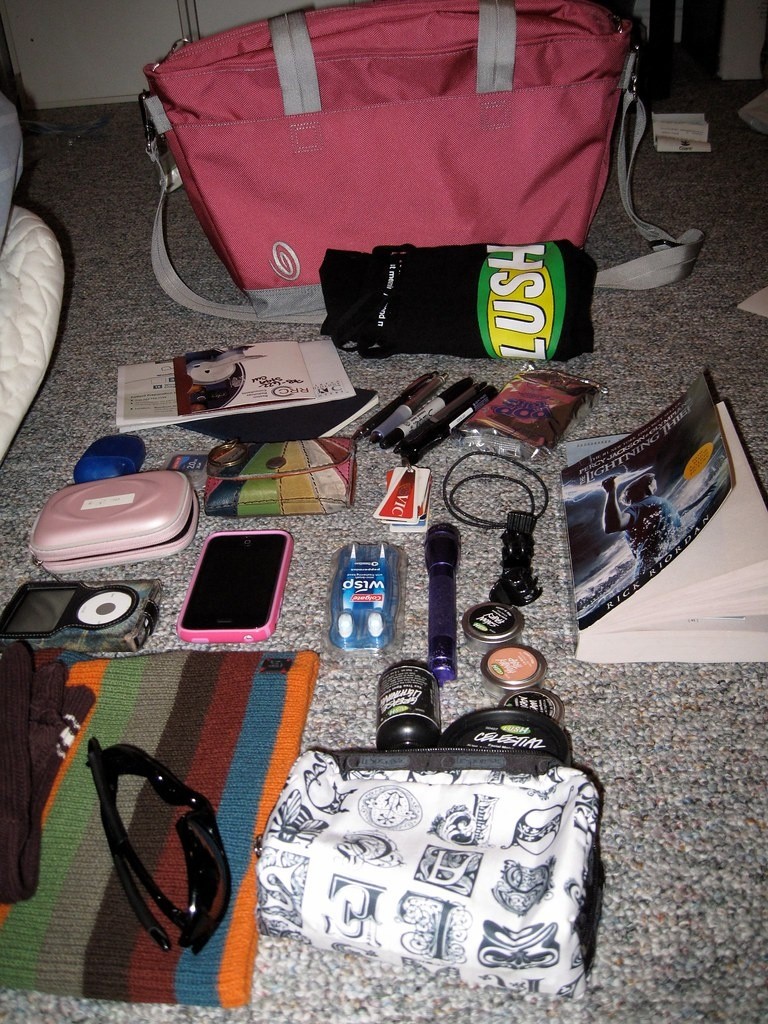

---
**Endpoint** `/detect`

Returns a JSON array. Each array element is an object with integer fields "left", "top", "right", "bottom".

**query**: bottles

[{"left": 377, "top": 660, "right": 440, "bottom": 753}]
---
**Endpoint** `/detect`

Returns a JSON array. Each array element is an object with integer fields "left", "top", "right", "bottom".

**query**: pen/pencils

[{"left": 352, "top": 369, "right": 487, "bottom": 454}]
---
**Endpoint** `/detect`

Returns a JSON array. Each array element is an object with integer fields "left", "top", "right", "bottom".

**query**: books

[
  {"left": 173, "top": 385, "right": 380, "bottom": 442},
  {"left": 560, "top": 372, "right": 768, "bottom": 663}
]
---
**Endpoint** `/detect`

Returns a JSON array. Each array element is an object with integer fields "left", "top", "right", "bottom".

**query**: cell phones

[{"left": 176, "top": 530, "right": 293, "bottom": 643}]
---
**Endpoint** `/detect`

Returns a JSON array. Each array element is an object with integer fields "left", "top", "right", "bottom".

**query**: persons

[{"left": 600, "top": 473, "right": 684, "bottom": 579}]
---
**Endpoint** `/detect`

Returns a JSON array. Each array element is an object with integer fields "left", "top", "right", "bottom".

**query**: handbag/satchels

[
  {"left": 138, "top": 0, "right": 706, "bottom": 326},
  {"left": 255, "top": 747, "right": 607, "bottom": 1003}
]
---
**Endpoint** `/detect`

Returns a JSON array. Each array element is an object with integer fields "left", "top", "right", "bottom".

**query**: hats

[{"left": 0, "top": 648, "right": 320, "bottom": 1008}]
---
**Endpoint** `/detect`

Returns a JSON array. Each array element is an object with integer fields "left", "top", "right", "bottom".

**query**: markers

[{"left": 403, "top": 384, "right": 498, "bottom": 465}]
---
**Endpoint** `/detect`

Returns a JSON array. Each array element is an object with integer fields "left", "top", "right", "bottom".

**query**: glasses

[{"left": 85, "top": 737, "right": 232, "bottom": 956}]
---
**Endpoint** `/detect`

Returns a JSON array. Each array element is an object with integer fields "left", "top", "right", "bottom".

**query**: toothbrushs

[{"left": 335, "top": 539, "right": 389, "bottom": 646}]
